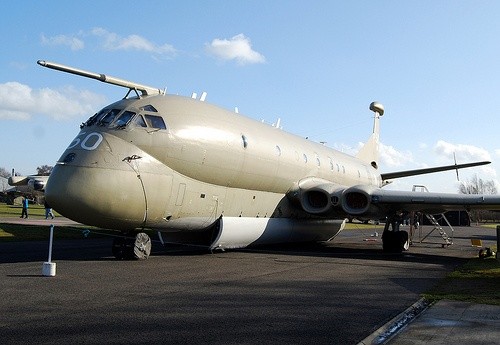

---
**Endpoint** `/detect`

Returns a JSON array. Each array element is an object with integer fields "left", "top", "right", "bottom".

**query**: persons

[
  {"left": 20, "top": 194, "right": 28, "bottom": 219},
  {"left": 44, "top": 197, "right": 55, "bottom": 219}
]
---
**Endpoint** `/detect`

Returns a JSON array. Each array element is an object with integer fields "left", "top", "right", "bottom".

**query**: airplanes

[{"left": 8, "top": 61, "right": 500, "bottom": 259}]
